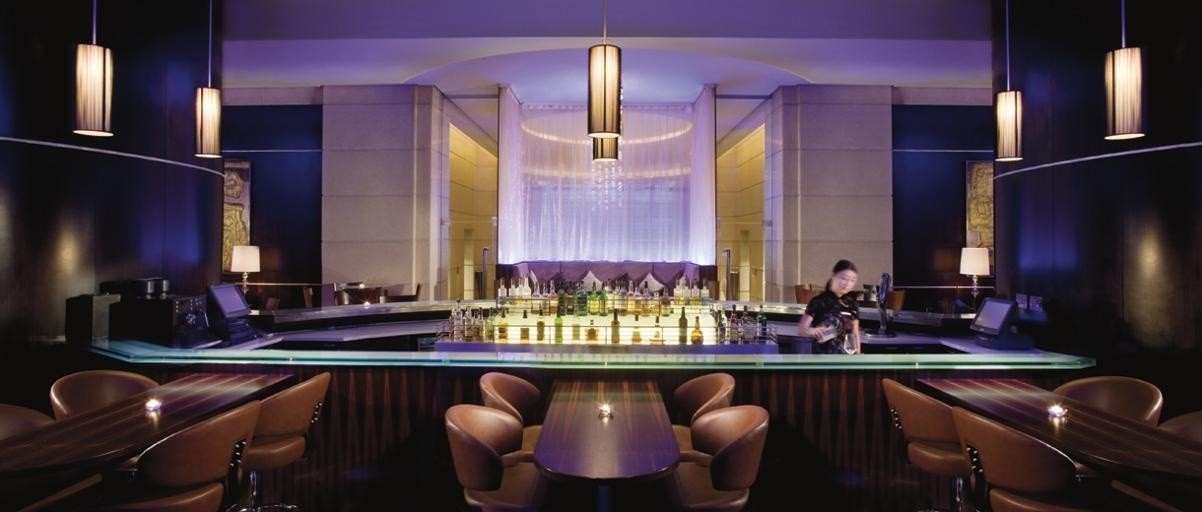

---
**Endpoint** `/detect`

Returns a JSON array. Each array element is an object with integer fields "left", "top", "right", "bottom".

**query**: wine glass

[{"left": 844, "top": 332, "right": 857, "bottom": 355}]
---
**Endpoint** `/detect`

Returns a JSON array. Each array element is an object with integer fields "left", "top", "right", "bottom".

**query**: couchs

[{"left": 512, "top": 261, "right": 701, "bottom": 297}]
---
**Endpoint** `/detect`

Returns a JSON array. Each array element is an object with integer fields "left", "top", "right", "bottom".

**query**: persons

[{"left": 797, "top": 259, "right": 863, "bottom": 355}]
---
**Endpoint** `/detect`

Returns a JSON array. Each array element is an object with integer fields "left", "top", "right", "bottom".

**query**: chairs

[
  {"left": 793, "top": 283, "right": 906, "bottom": 311},
  {"left": 301, "top": 283, "right": 423, "bottom": 308}
]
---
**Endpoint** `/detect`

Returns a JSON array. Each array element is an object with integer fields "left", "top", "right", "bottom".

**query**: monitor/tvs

[
  {"left": 207, "top": 284, "right": 251, "bottom": 327},
  {"left": 969, "top": 297, "right": 1018, "bottom": 343}
]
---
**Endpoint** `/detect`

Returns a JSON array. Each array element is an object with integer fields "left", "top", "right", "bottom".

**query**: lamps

[
  {"left": 1102, "top": 1, "right": 1149, "bottom": 143},
  {"left": 74, "top": 1, "right": 116, "bottom": 139},
  {"left": 586, "top": 0, "right": 623, "bottom": 162},
  {"left": 992, "top": 1, "right": 1024, "bottom": 163},
  {"left": 192, "top": 1, "right": 224, "bottom": 160}
]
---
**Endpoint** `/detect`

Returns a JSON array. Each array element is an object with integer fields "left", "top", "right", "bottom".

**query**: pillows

[{"left": 528, "top": 270, "right": 666, "bottom": 294}]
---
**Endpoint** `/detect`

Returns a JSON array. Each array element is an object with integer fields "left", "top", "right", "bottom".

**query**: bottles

[
  {"left": 815, "top": 315, "right": 854, "bottom": 344},
  {"left": 537, "top": 311, "right": 545, "bottom": 340},
  {"left": 587, "top": 321, "right": 599, "bottom": 340},
  {"left": 692, "top": 316, "right": 703, "bottom": 343},
  {"left": 651, "top": 318, "right": 662, "bottom": 341},
  {"left": 521, "top": 311, "right": 530, "bottom": 340},
  {"left": 611, "top": 309, "right": 620, "bottom": 343},
  {"left": 573, "top": 323, "right": 580, "bottom": 341},
  {"left": 449, "top": 300, "right": 497, "bottom": 344},
  {"left": 499, "top": 306, "right": 509, "bottom": 338},
  {"left": 497, "top": 275, "right": 713, "bottom": 316},
  {"left": 554, "top": 306, "right": 563, "bottom": 343},
  {"left": 678, "top": 307, "right": 687, "bottom": 345},
  {"left": 631, "top": 315, "right": 643, "bottom": 343},
  {"left": 714, "top": 304, "right": 771, "bottom": 344}
]
